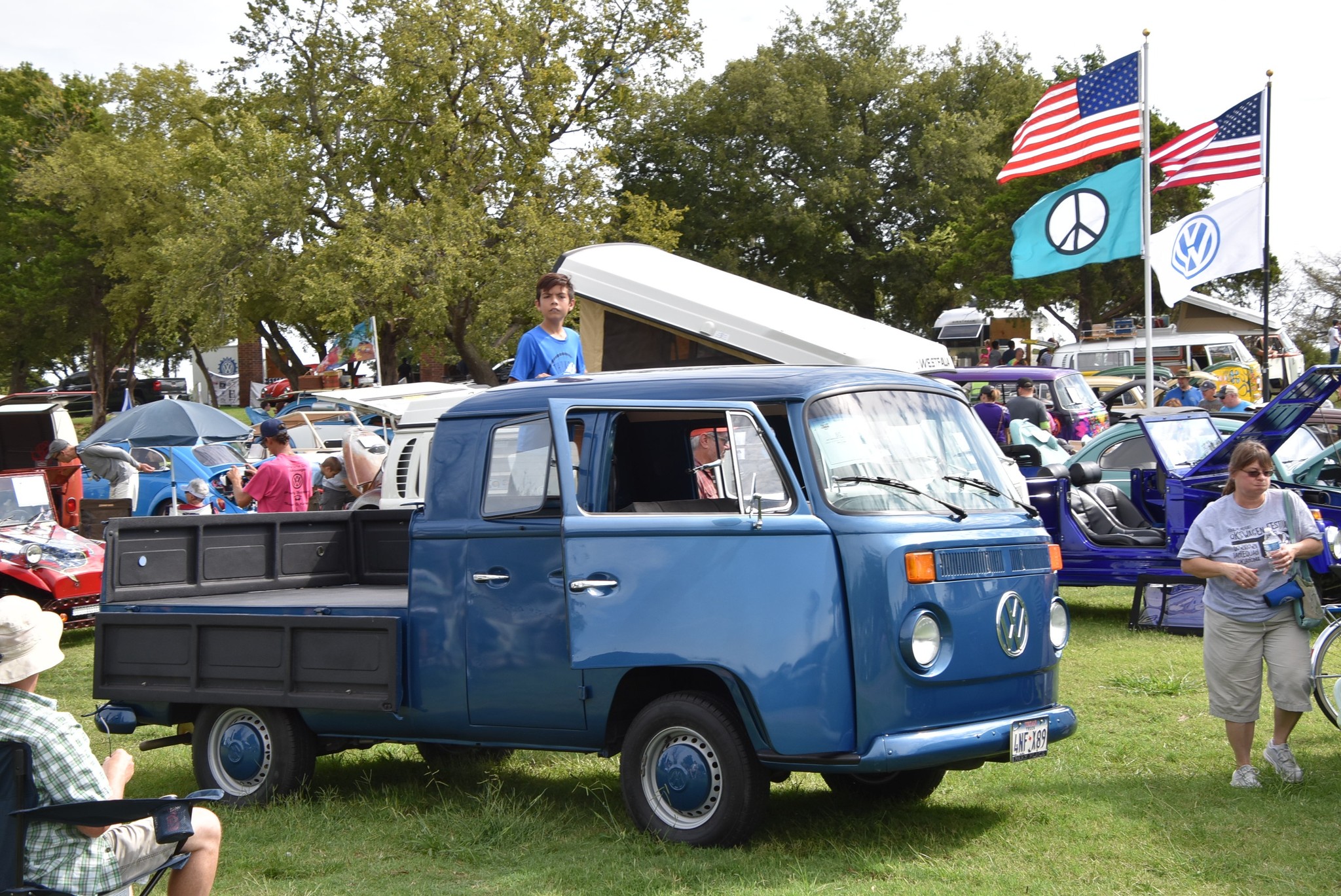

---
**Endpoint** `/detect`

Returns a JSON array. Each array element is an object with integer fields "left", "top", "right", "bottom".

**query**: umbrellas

[{"left": 77, "top": 399, "right": 255, "bottom": 513}]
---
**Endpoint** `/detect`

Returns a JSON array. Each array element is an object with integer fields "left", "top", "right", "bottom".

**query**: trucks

[{"left": 96, "top": 367, "right": 1078, "bottom": 849}]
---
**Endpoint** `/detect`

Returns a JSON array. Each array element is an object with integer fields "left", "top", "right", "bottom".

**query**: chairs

[
  {"left": 1035, "top": 462, "right": 1163, "bottom": 548},
  {"left": 0, "top": 737, "right": 226, "bottom": 896},
  {"left": 1068, "top": 460, "right": 1165, "bottom": 535}
]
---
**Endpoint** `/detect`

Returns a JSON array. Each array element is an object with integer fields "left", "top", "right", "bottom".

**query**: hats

[
  {"left": 179, "top": 484, "right": 207, "bottom": 499},
  {"left": 1196, "top": 380, "right": 1216, "bottom": 391},
  {"left": 975, "top": 385, "right": 995, "bottom": 398},
  {"left": 44, "top": 439, "right": 68, "bottom": 460},
  {"left": 1171, "top": 369, "right": 1194, "bottom": 379},
  {"left": 254, "top": 418, "right": 286, "bottom": 444},
  {"left": 0, "top": 593, "right": 65, "bottom": 683},
  {"left": 690, "top": 427, "right": 737, "bottom": 436},
  {"left": 1213, "top": 384, "right": 1239, "bottom": 397},
  {"left": 1332, "top": 319, "right": 1341, "bottom": 324},
  {"left": 1016, "top": 377, "right": 1033, "bottom": 388}
]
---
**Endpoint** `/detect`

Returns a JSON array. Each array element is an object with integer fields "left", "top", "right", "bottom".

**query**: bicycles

[{"left": 1312, "top": 604, "right": 1341, "bottom": 729}]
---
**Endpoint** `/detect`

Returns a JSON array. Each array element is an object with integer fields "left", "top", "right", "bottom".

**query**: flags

[
  {"left": 315, "top": 318, "right": 376, "bottom": 373},
  {"left": 1011, "top": 156, "right": 1144, "bottom": 279},
  {"left": 997, "top": 52, "right": 1144, "bottom": 188},
  {"left": 1150, "top": 86, "right": 1268, "bottom": 191},
  {"left": 1150, "top": 188, "right": 1265, "bottom": 307}
]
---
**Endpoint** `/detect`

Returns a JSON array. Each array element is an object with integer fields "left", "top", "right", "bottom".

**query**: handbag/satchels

[{"left": 1290, "top": 574, "right": 1325, "bottom": 627}]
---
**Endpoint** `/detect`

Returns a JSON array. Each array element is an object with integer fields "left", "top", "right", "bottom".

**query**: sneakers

[
  {"left": 1264, "top": 739, "right": 1304, "bottom": 783},
  {"left": 1229, "top": 765, "right": 1264, "bottom": 788}
]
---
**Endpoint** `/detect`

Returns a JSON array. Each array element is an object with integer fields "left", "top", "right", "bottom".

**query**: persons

[
  {"left": 170, "top": 478, "right": 219, "bottom": 516},
  {"left": 1250, "top": 338, "right": 1278, "bottom": 368},
  {"left": 1036, "top": 338, "right": 1060, "bottom": 367},
  {"left": 311, "top": 452, "right": 356, "bottom": 511},
  {"left": 1159, "top": 369, "right": 1255, "bottom": 415},
  {"left": 1177, "top": 436, "right": 1326, "bottom": 788},
  {"left": 971, "top": 377, "right": 1056, "bottom": 447},
  {"left": 0, "top": 594, "right": 219, "bottom": 896},
  {"left": 505, "top": 273, "right": 586, "bottom": 498},
  {"left": 398, "top": 357, "right": 410, "bottom": 384},
  {"left": 976, "top": 339, "right": 1029, "bottom": 366},
  {"left": 690, "top": 428, "right": 730, "bottom": 499},
  {"left": 260, "top": 401, "right": 271, "bottom": 417},
  {"left": 1306, "top": 384, "right": 1335, "bottom": 409},
  {"left": 45, "top": 439, "right": 155, "bottom": 511},
  {"left": 225, "top": 419, "right": 312, "bottom": 513},
  {"left": 1328, "top": 320, "right": 1341, "bottom": 365}
]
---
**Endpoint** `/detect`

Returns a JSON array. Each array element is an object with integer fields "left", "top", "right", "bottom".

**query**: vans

[
  {"left": 1053, "top": 325, "right": 1304, "bottom": 403},
  {"left": 0, "top": 391, "right": 96, "bottom": 532}
]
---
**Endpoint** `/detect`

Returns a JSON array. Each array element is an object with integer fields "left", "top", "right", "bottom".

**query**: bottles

[{"left": 1263, "top": 527, "right": 1286, "bottom": 572}]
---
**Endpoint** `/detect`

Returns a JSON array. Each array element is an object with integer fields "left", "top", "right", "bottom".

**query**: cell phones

[{"left": 236, "top": 466, "right": 249, "bottom": 477}]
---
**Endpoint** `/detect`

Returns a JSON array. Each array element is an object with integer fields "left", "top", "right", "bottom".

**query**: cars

[
  {"left": 0, "top": 383, "right": 522, "bottom": 630},
  {"left": 256, "top": 363, "right": 320, "bottom": 404},
  {"left": 912, "top": 361, "right": 1341, "bottom": 606}
]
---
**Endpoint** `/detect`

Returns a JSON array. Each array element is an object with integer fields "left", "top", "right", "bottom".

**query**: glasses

[
  {"left": 184, "top": 491, "right": 191, "bottom": 494},
  {"left": 260, "top": 439, "right": 267, "bottom": 449},
  {"left": 53, "top": 452, "right": 59, "bottom": 460},
  {"left": 97, "top": 717, "right": 111, "bottom": 765},
  {"left": 709, "top": 435, "right": 728, "bottom": 445},
  {"left": 1214, "top": 394, "right": 1232, "bottom": 399},
  {"left": 1240, "top": 468, "right": 1273, "bottom": 477}
]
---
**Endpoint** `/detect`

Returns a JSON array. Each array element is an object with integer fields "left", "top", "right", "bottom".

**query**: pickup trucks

[{"left": 29, "top": 369, "right": 191, "bottom": 412}]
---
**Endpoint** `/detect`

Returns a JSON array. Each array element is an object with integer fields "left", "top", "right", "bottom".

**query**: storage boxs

[
  {"left": 1091, "top": 322, "right": 1108, "bottom": 337},
  {"left": 1111, "top": 316, "right": 1134, "bottom": 334}
]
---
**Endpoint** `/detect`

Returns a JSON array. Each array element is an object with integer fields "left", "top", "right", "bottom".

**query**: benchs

[{"left": 619, "top": 493, "right": 744, "bottom": 516}]
